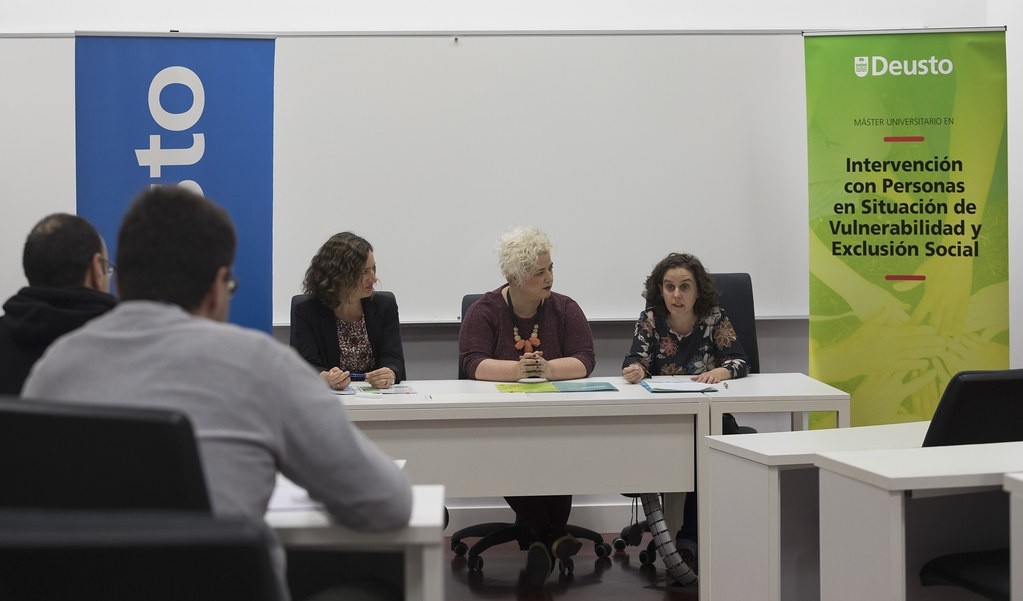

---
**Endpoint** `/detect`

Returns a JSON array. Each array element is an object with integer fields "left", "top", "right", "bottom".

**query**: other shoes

[
  {"left": 676, "top": 531, "right": 697, "bottom": 570},
  {"left": 524, "top": 542, "right": 553, "bottom": 588},
  {"left": 545, "top": 522, "right": 583, "bottom": 558}
]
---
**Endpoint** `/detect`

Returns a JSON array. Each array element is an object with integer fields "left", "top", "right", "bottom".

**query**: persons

[
  {"left": 0, "top": 214, "right": 118, "bottom": 397},
  {"left": 622, "top": 253, "right": 751, "bottom": 576},
  {"left": 18, "top": 186, "right": 413, "bottom": 601},
  {"left": 459, "top": 228, "right": 596, "bottom": 587},
  {"left": 295, "top": 231, "right": 404, "bottom": 390}
]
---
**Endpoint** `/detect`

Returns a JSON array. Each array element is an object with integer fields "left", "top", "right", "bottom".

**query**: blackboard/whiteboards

[{"left": 0, "top": 29, "right": 810, "bottom": 335}]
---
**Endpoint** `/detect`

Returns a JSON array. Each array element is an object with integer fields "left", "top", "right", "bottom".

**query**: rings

[
  {"left": 385, "top": 379, "right": 388, "bottom": 385},
  {"left": 338, "top": 384, "right": 344, "bottom": 390}
]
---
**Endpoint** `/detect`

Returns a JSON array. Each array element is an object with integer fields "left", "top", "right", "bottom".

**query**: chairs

[
  {"left": 290, "top": 291, "right": 406, "bottom": 384},
  {"left": 922, "top": 369, "right": 1023, "bottom": 447},
  {"left": 0, "top": 395, "right": 212, "bottom": 513},
  {"left": 612, "top": 272, "right": 759, "bottom": 565},
  {"left": 451, "top": 294, "right": 612, "bottom": 584}
]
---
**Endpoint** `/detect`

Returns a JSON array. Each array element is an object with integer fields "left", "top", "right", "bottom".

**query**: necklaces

[{"left": 507, "top": 287, "right": 543, "bottom": 354}]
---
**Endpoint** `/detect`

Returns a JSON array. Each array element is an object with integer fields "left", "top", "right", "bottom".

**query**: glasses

[
  {"left": 229, "top": 268, "right": 239, "bottom": 298},
  {"left": 100, "top": 258, "right": 115, "bottom": 278}
]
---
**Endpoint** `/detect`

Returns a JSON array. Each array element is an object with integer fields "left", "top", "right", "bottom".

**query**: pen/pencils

[
  {"left": 348, "top": 374, "right": 366, "bottom": 378},
  {"left": 724, "top": 382, "right": 728, "bottom": 390}
]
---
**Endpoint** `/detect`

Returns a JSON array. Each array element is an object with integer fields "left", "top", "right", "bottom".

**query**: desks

[
  {"left": 703, "top": 421, "right": 933, "bottom": 601},
  {"left": 330, "top": 372, "right": 850, "bottom": 497},
  {"left": 263, "top": 484, "right": 445, "bottom": 601},
  {"left": 813, "top": 442, "right": 1023, "bottom": 601}
]
